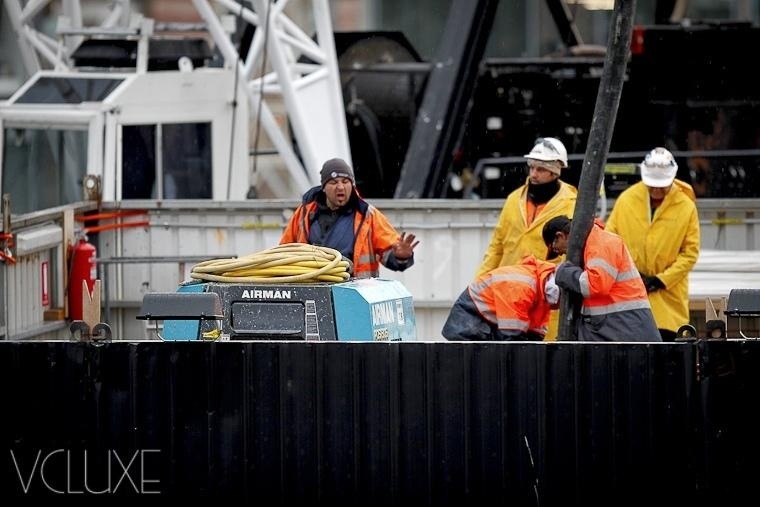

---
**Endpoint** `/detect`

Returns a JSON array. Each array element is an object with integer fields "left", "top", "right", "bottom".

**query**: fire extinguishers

[{"left": 68, "top": 229, "right": 97, "bottom": 321}]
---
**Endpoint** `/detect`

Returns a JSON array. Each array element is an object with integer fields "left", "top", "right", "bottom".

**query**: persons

[
  {"left": 542, "top": 213, "right": 665, "bottom": 342},
  {"left": 277, "top": 156, "right": 421, "bottom": 278},
  {"left": 439, "top": 253, "right": 567, "bottom": 341},
  {"left": 603, "top": 145, "right": 702, "bottom": 340},
  {"left": 471, "top": 135, "right": 578, "bottom": 341},
  {"left": 687, "top": 101, "right": 733, "bottom": 198}
]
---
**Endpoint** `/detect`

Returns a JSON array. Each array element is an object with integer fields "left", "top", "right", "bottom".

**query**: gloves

[{"left": 639, "top": 271, "right": 664, "bottom": 293}]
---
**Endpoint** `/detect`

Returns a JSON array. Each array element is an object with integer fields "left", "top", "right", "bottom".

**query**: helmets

[
  {"left": 640, "top": 147, "right": 679, "bottom": 189},
  {"left": 523, "top": 138, "right": 569, "bottom": 169}
]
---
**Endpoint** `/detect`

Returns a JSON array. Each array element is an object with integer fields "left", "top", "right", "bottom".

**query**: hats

[
  {"left": 320, "top": 158, "right": 354, "bottom": 190},
  {"left": 542, "top": 215, "right": 571, "bottom": 261}
]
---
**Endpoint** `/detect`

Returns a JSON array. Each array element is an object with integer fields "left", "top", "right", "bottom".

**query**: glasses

[
  {"left": 644, "top": 161, "right": 671, "bottom": 169},
  {"left": 535, "top": 137, "right": 561, "bottom": 155}
]
